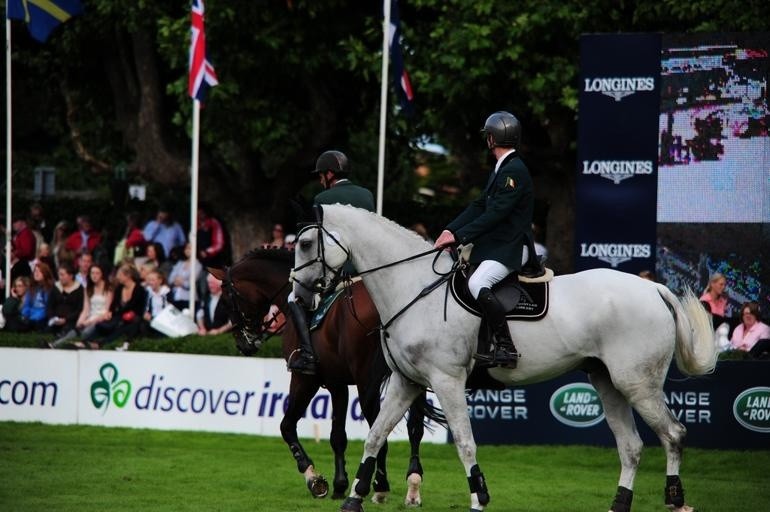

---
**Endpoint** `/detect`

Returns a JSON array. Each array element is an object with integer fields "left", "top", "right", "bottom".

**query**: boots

[
  {"left": 286, "top": 299, "right": 321, "bottom": 376},
  {"left": 471, "top": 286, "right": 522, "bottom": 369}
]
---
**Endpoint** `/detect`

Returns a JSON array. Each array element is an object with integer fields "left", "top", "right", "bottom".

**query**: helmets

[
  {"left": 478, "top": 110, "right": 523, "bottom": 150},
  {"left": 311, "top": 149, "right": 352, "bottom": 176}
]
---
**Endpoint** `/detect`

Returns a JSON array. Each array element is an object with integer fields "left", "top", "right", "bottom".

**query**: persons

[
  {"left": 287, "top": 150, "right": 376, "bottom": 374},
  {"left": 436, "top": 112, "right": 538, "bottom": 369}
]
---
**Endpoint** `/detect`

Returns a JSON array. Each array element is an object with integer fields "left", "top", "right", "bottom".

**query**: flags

[
  {"left": 386, "top": 0, "right": 413, "bottom": 112},
  {"left": 8, "top": 0, "right": 83, "bottom": 42},
  {"left": 189, "top": 0, "right": 220, "bottom": 109}
]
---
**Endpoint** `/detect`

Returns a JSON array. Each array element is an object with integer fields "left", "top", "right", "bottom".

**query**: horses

[
  {"left": 204, "top": 242, "right": 448, "bottom": 507},
  {"left": 286, "top": 199, "right": 719, "bottom": 512}
]
